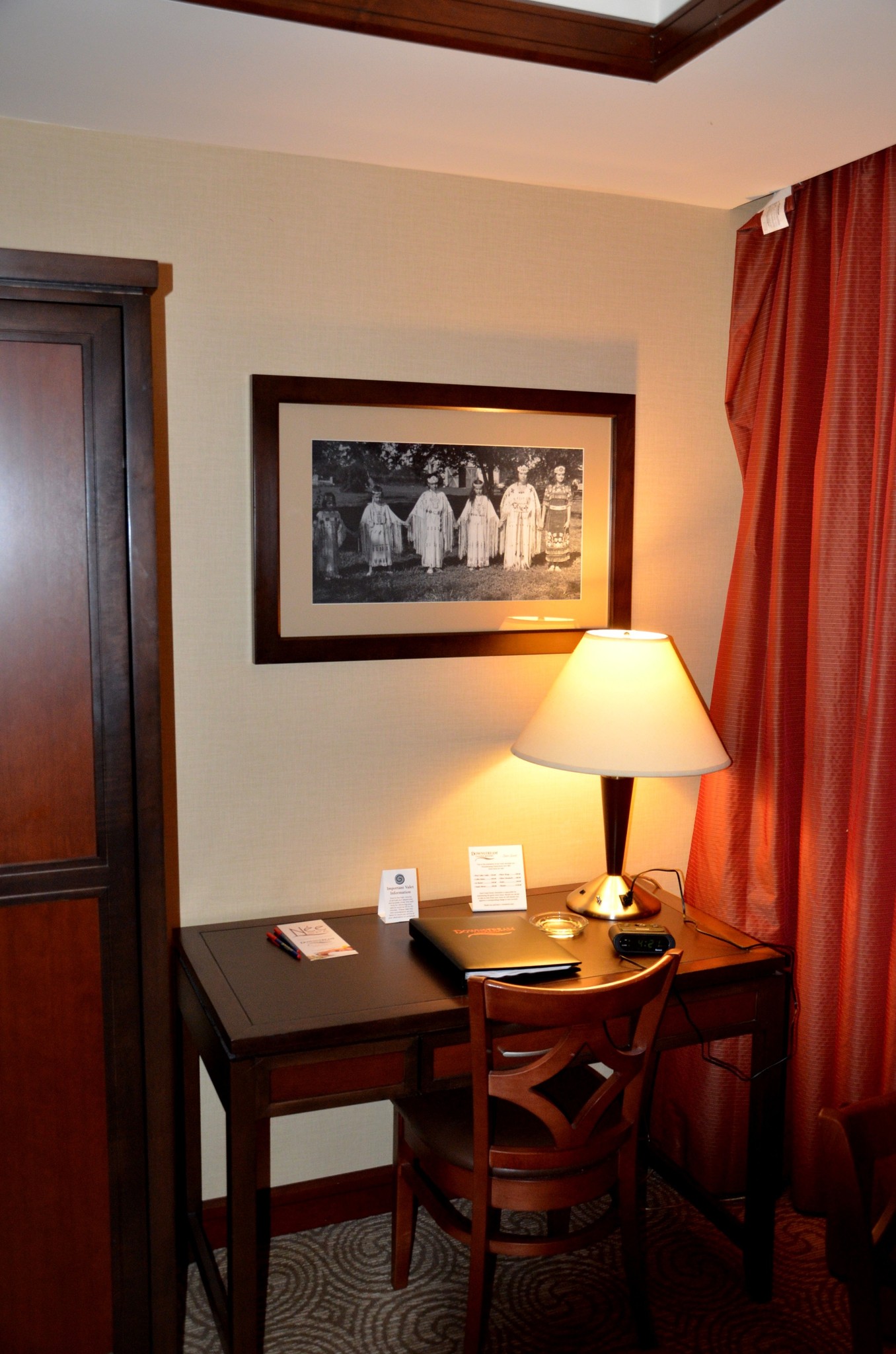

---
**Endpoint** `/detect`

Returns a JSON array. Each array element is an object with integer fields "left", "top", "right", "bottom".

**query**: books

[{"left": 409, "top": 915, "right": 581, "bottom": 986}]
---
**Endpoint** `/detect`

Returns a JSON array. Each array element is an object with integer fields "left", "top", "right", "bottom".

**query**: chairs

[
  {"left": 388, "top": 945, "right": 684, "bottom": 1354},
  {"left": 818, "top": 1092, "right": 896, "bottom": 1354}
]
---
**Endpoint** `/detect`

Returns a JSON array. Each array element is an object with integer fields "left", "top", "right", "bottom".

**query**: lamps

[{"left": 510, "top": 628, "right": 732, "bottom": 920}]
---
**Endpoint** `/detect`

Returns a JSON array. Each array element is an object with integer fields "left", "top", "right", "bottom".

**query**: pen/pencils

[
  {"left": 273, "top": 927, "right": 300, "bottom": 954},
  {"left": 266, "top": 931, "right": 301, "bottom": 960}
]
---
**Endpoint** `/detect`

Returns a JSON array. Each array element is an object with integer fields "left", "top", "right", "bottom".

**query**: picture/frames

[{"left": 251, "top": 374, "right": 635, "bottom": 665}]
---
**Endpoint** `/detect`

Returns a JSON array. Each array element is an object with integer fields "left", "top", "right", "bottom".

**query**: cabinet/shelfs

[{"left": 0, "top": 245, "right": 194, "bottom": 1354}]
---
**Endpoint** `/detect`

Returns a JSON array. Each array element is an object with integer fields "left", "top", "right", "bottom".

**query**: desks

[{"left": 174, "top": 877, "right": 789, "bottom": 1354}]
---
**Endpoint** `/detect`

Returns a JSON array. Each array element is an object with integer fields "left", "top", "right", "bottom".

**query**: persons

[
  {"left": 405, "top": 473, "right": 460, "bottom": 575},
  {"left": 454, "top": 480, "right": 503, "bottom": 571},
  {"left": 312, "top": 493, "right": 356, "bottom": 580},
  {"left": 499, "top": 464, "right": 543, "bottom": 571},
  {"left": 542, "top": 466, "right": 573, "bottom": 572},
  {"left": 355, "top": 486, "right": 410, "bottom": 577}
]
